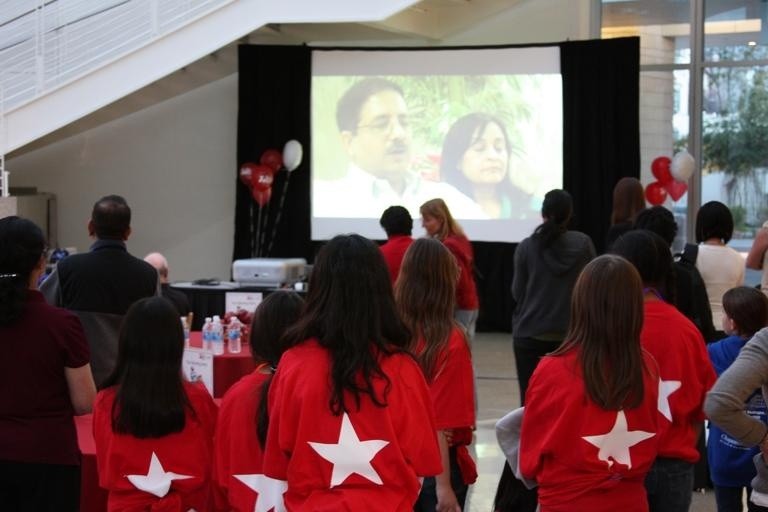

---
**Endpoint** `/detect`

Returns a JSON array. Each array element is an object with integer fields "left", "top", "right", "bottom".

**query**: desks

[{"left": 167, "top": 278, "right": 310, "bottom": 332}]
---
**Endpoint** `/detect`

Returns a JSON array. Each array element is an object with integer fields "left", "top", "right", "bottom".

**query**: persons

[
  {"left": 509, "top": 188, "right": 597, "bottom": 407},
  {"left": 635, "top": 205, "right": 678, "bottom": 246},
  {"left": 611, "top": 232, "right": 717, "bottom": 511},
  {"left": 91, "top": 297, "right": 215, "bottom": 512},
  {"left": 606, "top": 176, "right": 646, "bottom": 249},
  {"left": 379, "top": 197, "right": 478, "bottom": 347},
  {"left": 675, "top": 200, "right": 745, "bottom": 333},
  {"left": 214, "top": 289, "right": 311, "bottom": 510},
  {"left": 57, "top": 194, "right": 159, "bottom": 392},
  {"left": 745, "top": 221, "right": 768, "bottom": 295},
  {"left": 266, "top": 234, "right": 439, "bottom": 511},
  {"left": 703, "top": 322, "right": 767, "bottom": 512},
  {"left": 521, "top": 253, "right": 668, "bottom": 510},
  {"left": 311, "top": 82, "right": 479, "bottom": 239},
  {"left": 3, "top": 217, "right": 97, "bottom": 512},
  {"left": 438, "top": 114, "right": 548, "bottom": 239},
  {"left": 703, "top": 286, "right": 768, "bottom": 511},
  {"left": 391, "top": 237, "right": 477, "bottom": 511},
  {"left": 144, "top": 250, "right": 192, "bottom": 319}
]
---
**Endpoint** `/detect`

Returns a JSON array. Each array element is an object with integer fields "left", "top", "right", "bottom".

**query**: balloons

[
  {"left": 238, "top": 138, "right": 301, "bottom": 212},
  {"left": 645, "top": 148, "right": 697, "bottom": 206}
]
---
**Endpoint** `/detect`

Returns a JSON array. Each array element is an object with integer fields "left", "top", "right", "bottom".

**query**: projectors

[{"left": 233, "top": 258, "right": 306, "bottom": 284}]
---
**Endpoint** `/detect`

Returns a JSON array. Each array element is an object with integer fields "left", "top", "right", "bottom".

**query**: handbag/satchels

[{"left": 645, "top": 460, "right": 693, "bottom": 511}]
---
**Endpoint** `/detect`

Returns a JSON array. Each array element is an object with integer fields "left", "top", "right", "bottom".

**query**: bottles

[{"left": 180, "top": 315, "right": 243, "bottom": 356}]
